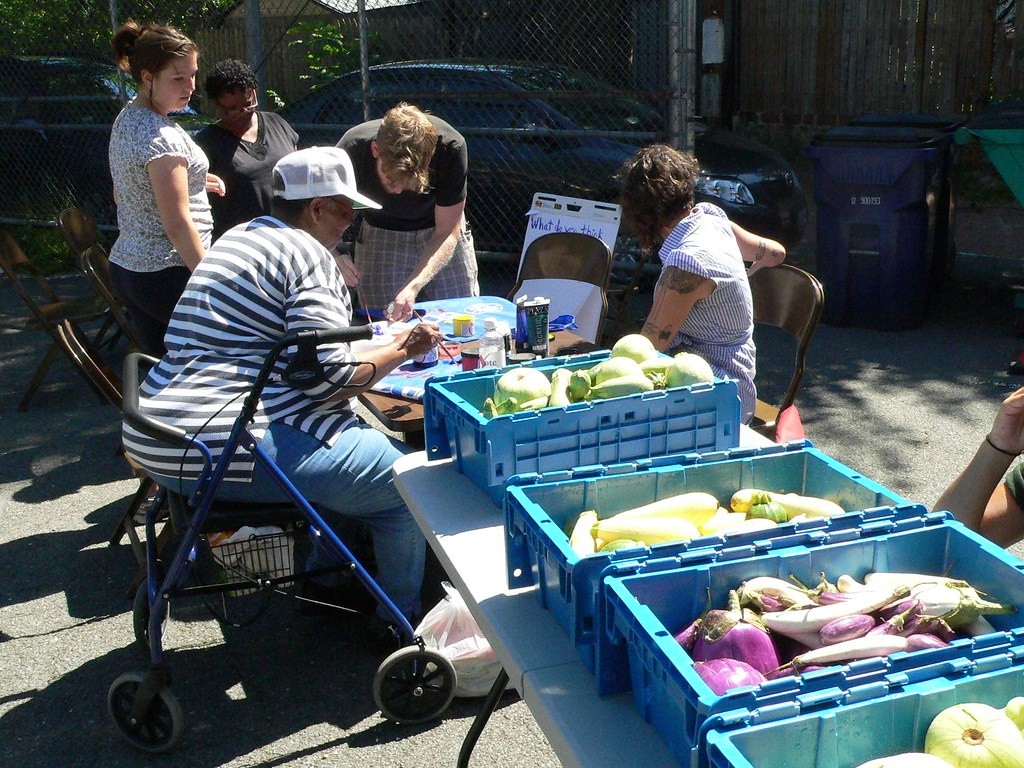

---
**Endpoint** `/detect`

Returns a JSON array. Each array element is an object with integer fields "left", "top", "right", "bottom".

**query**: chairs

[
  {"left": 506, "top": 232, "right": 612, "bottom": 346},
  {"left": 0, "top": 206, "right": 166, "bottom": 413},
  {"left": 740, "top": 260, "right": 825, "bottom": 440}
]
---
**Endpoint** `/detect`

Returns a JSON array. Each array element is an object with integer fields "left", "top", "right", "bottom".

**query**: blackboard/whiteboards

[{"left": 515, "top": 192, "right": 623, "bottom": 285}]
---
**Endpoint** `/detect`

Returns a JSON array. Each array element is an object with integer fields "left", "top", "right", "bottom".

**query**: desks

[{"left": 330, "top": 296, "right": 783, "bottom": 768}]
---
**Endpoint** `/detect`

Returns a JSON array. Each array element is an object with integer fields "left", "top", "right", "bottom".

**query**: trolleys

[{"left": 58, "top": 317, "right": 458, "bottom": 751}]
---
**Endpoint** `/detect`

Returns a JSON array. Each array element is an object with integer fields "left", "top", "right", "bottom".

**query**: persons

[
  {"left": 327, "top": 102, "right": 480, "bottom": 323},
  {"left": 194, "top": 60, "right": 299, "bottom": 244},
  {"left": 931, "top": 386, "right": 1024, "bottom": 550},
  {"left": 123, "top": 145, "right": 442, "bottom": 645},
  {"left": 615, "top": 143, "right": 785, "bottom": 425},
  {"left": 106, "top": 17, "right": 227, "bottom": 526}
]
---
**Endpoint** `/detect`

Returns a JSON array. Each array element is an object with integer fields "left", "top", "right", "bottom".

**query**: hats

[{"left": 271, "top": 145, "right": 383, "bottom": 211}]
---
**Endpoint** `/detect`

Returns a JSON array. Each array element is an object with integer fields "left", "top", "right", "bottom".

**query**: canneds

[{"left": 413, "top": 346, "right": 439, "bottom": 368}]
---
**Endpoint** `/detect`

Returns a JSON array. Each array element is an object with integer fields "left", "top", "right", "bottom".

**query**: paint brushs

[
  {"left": 414, "top": 310, "right": 463, "bottom": 370},
  {"left": 358, "top": 284, "right": 372, "bottom": 325}
]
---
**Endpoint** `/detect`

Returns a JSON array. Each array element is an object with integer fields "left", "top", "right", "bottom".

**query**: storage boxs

[
  {"left": 504, "top": 440, "right": 928, "bottom": 647},
  {"left": 705, "top": 664, "right": 1024, "bottom": 768},
  {"left": 589, "top": 512, "right": 1023, "bottom": 765},
  {"left": 422, "top": 345, "right": 742, "bottom": 506}
]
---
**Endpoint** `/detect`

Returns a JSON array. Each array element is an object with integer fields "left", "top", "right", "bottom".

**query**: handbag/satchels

[{"left": 415, "top": 580, "right": 517, "bottom": 701}]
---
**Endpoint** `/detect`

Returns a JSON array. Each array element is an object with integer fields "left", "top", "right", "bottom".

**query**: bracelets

[{"left": 986, "top": 435, "right": 1022, "bottom": 456}]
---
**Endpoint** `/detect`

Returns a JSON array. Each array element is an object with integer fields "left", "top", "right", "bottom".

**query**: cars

[
  {"left": 0, "top": 56, "right": 209, "bottom": 215},
  {"left": 273, "top": 58, "right": 809, "bottom": 283}
]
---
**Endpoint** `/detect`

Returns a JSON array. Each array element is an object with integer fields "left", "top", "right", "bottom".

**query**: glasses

[{"left": 212, "top": 89, "right": 259, "bottom": 118}]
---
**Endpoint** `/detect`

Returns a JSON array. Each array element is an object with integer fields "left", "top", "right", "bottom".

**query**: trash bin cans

[{"left": 802, "top": 115, "right": 962, "bottom": 330}]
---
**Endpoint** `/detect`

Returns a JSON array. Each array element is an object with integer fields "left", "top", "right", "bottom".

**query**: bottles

[
  {"left": 461, "top": 349, "right": 480, "bottom": 372},
  {"left": 453, "top": 315, "right": 475, "bottom": 336},
  {"left": 414, "top": 346, "right": 438, "bottom": 368},
  {"left": 509, "top": 353, "right": 536, "bottom": 364},
  {"left": 477, "top": 318, "right": 506, "bottom": 369},
  {"left": 509, "top": 328, "right": 517, "bottom": 353},
  {"left": 497, "top": 321, "right": 511, "bottom": 357}
]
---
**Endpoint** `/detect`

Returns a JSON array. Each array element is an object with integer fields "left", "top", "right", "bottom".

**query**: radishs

[{"left": 569, "top": 488, "right": 845, "bottom": 554}]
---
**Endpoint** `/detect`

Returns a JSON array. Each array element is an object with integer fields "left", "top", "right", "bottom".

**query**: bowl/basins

[{"left": 438, "top": 341, "right": 461, "bottom": 356}]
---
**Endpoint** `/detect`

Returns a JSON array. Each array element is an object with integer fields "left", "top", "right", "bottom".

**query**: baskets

[{"left": 206, "top": 528, "right": 298, "bottom": 598}]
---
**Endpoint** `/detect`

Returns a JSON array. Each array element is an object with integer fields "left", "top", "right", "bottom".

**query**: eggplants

[{"left": 675, "top": 572, "right": 1018, "bottom": 695}]
